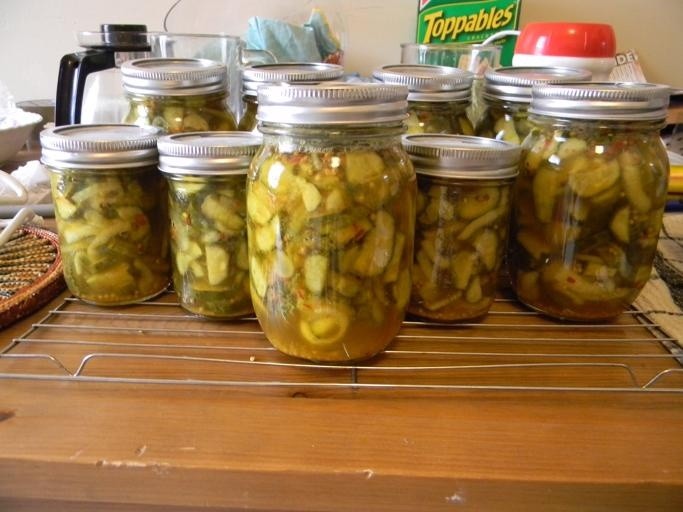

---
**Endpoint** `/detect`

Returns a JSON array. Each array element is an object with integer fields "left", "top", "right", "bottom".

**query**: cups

[
  {"left": 397, "top": 42, "right": 503, "bottom": 128},
  {"left": 50, "top": 22, "right": 152, "bottom": 127},
  {"left": 149, "top": 32, "right": 279, "bottom": 127}
]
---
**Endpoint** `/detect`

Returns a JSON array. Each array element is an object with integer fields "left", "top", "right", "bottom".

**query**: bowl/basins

[{"left": 0, "top": 111, "right": 44, "bottom": 166}]
